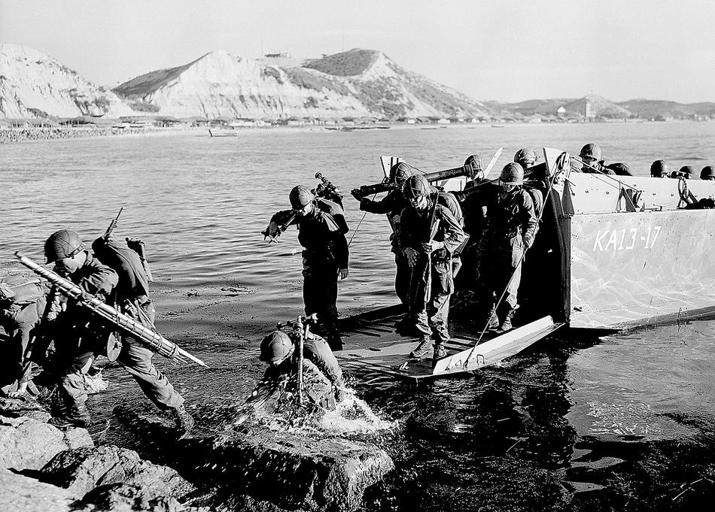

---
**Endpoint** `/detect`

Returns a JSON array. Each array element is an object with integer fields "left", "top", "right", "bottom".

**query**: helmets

[
  {"left": 290, "top": 185, "right": 315, "bottom": 209},
  {"left": 390, "top": 162, "right": 430, "bottom": 198},
  {"left": 259, "top": 331, "right": 292, "bottom": 361},
  {"left": 465, "top": 155, "right": 483, "bottom": 170},
  {"left": 651, "top": 160, "right": 715, "bottom": 179},
  {"left": 0, "top": 283, "right": 16, "bottom": 300},
  {"left": 501, "top": 148, "right": 538, "bottom": 185},
  {"left": 44, "top": 230, "right": 86, "bottom": 265},
  {"left": 579, "top": 143, "right": 601, "bottom": 161}
]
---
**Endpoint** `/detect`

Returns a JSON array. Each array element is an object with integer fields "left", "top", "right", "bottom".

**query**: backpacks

[{"left": 433, "top": 192, "right": 464, "bottom": 229}]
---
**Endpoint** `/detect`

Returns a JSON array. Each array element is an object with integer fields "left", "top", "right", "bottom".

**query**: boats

[
  {"left": 208, "top": 128, "right": 240, "bottom": 138},
  {"left": 317, "top": 171, "right": 715, "bottom": 387}
]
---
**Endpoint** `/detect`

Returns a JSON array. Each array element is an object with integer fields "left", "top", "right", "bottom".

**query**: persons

[
  {"left": 0, "top": 281, "right": 62, "bottom": 399},
  {"left": 248, "top": 331, "right": 336, "bottom": 422},
  {"left": 260, "top": 185, "right": 349, "bottom": 352},
  {"left": 45, "top": 230, "right": 194, "bottom": 442}
]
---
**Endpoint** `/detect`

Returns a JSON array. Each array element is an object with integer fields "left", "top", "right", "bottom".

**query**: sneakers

[
  {"left": 170, "top": 413, "right": 194, "bottom": 441},
  {"left": 410, "top": 340, "right": 447, "bottom": 360},
  {"left": 484, "top": 314, "right": 512, "bottom": 331},
  {"left": 48, "top": 414, "right": 90, "bottom": 427}
]
